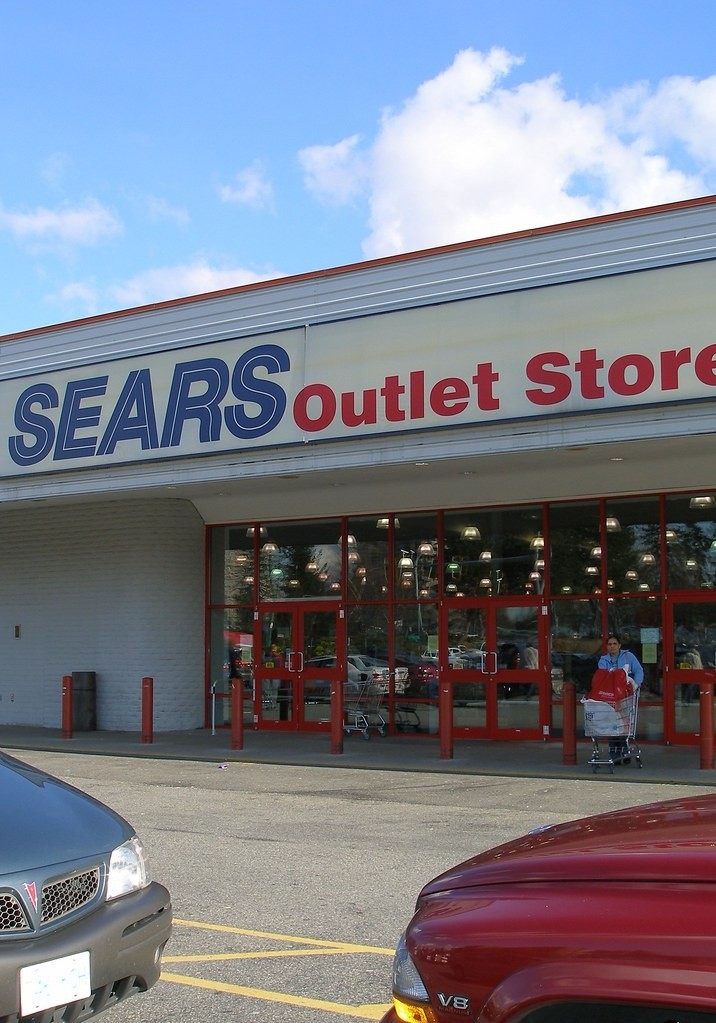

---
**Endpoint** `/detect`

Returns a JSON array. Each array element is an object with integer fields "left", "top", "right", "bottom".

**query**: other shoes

[
  {"left": 622, "top": 750, "right": 631, "bottom": 764},
  {"left": 608, "top": 754, "right": 621, "bottom": 765}
]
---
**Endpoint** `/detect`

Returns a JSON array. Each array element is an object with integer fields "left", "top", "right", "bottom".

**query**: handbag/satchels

[{"left": 586, "top": 664, "right": 638, "bottom": 734}]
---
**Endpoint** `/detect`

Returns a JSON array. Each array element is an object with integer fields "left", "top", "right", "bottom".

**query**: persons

[
  {"left": 261, "top": 643, "right": 283, "bottom": 710},
  {"left": 597, "top": 634, "right": 644, "bottom": 767},
  {"left": 682, "top": 649, "right": 704, "bottom": 704},
  {"left": 521, "top": 638, "right": 568, "bottom": 702}
]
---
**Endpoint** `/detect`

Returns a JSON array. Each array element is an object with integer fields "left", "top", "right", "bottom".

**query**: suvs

[{"left": 0, "top": 748, "right": 174, "bottom": 1023}]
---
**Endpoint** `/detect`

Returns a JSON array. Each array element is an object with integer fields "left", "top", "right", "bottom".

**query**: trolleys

[
  {"left": 341, "top": 668, "right": 388, "bottom": 740},
  {"left": 381, "top": 671, "right": 421, "bottom": 734},
  {"left": 582, "top": 686, "right": 646, "bottom": 775}
]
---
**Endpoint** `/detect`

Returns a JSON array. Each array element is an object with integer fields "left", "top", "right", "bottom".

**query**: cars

[
  {"left": 380, "top": 793, "right": 716, "bottom": 1023},
  {"left": 302, "top": 647, "right": 488, "bottom": 698}
]
